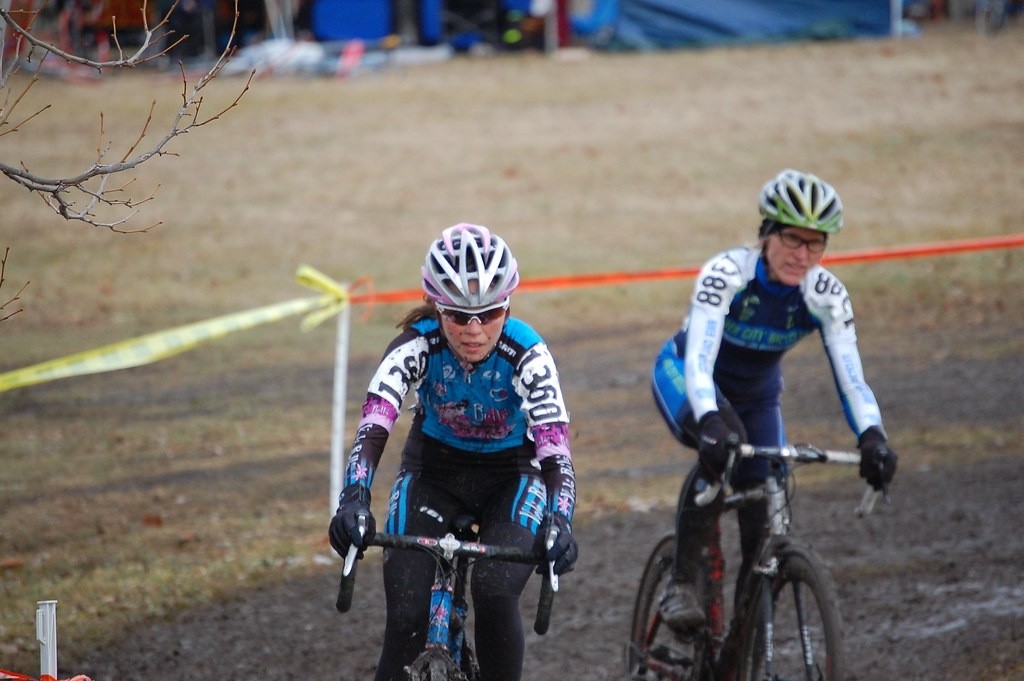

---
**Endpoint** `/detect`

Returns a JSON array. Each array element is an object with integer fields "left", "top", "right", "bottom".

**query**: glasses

[
  {"left": 775, "top": 229, "right": 826, "bottom": 253},
  {"left": 432, "top": 308, "right": 507, "bottom": 326}
]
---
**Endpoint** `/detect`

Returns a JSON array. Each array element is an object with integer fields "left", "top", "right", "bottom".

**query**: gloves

[
  {"left": 327, "top": 481, "right": 377, "bottom": 561},
  {"left": 532, "top": 511, "right": 578, "bottom": 577},
  {"left": 858, "top": 425, "right": 897, "bottom": 490},
  {"left": 695, "top": 410, "right": 739, "bottom": 484}
]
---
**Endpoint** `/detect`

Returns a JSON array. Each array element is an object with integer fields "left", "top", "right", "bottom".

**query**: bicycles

[
  {"left": 338, "top": 508, "right": 559, "bottom": 681},
  {"left": 628, "top": 431, "right": 889, "bottom": 681}
]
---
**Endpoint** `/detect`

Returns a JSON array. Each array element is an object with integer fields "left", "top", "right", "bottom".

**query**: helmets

[
  {"left": 759, "top": 169, "right": 842, "bottom": 233},
  {"left": 420, "top": 223, "right": 520, "bottom": 308}
]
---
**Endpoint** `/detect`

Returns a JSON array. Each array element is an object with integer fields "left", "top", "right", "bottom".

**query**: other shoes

[{"left": 659, "top": 582, "right": 706, "bottom": 628}]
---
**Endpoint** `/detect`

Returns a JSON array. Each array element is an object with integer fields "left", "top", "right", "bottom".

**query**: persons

[
  {"left": 328, "top": 223, "right": 579, "bottom": 680},
  {"left": 653, "top": 169, "right": 898, "bottom": 680}
]
